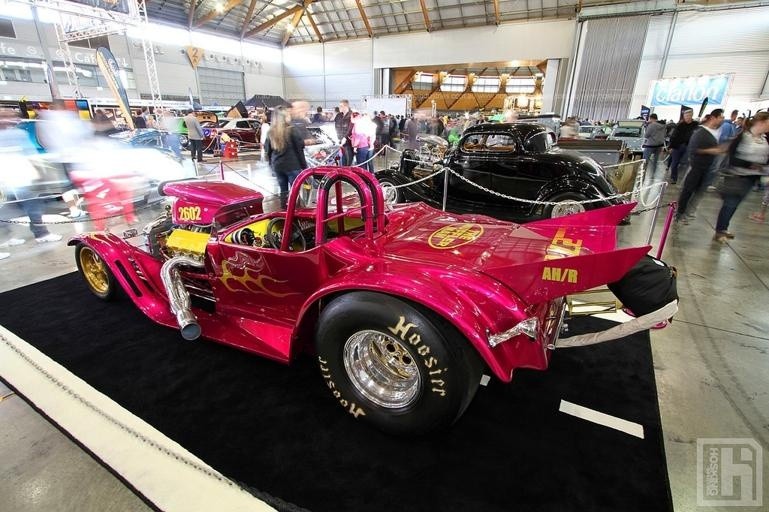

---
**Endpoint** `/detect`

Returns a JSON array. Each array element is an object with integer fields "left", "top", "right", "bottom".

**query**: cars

[
  {"left": 172, "top": 111, "right": 344, "bottom": 167},
  {"left": 578, "top": 121, "right": 645, "bottom": 152},
  {"left": 373, "top": 123, "right": 631, "bottom": 228}
]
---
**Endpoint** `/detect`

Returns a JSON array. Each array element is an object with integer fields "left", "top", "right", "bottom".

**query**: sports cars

[{"left": 67, "top": 168, "right": 679, "bottom": 438}]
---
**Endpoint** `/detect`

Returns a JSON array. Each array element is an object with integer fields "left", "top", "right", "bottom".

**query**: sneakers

[
  {"left": 674, "top": 212, "right": 696, "bottom": 224},
  {"left": 712, "top": 230, "right": 736, "bottom": 245},
  {"left": 1, "top": 233, "right": 62, "bottom": 262}
]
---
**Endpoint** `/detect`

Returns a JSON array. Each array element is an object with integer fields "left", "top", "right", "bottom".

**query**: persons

[
  {"left": 559, "top": 116, "right": 615, "bottom": 140},
  {"left": 184, "top": 109, "right": 207, "bottom": 162},
  {"left": 131, "top": 106, "right": 182, "bottom": 164},
  {"left": 641, "top": 108, "right": 769, "bottom": 244},
  {"left": 248, "top": 99, "right": 519, "bottom": 212},
  {"left": 0, "top": 103, "right": 117, "bottom": 260}
]
